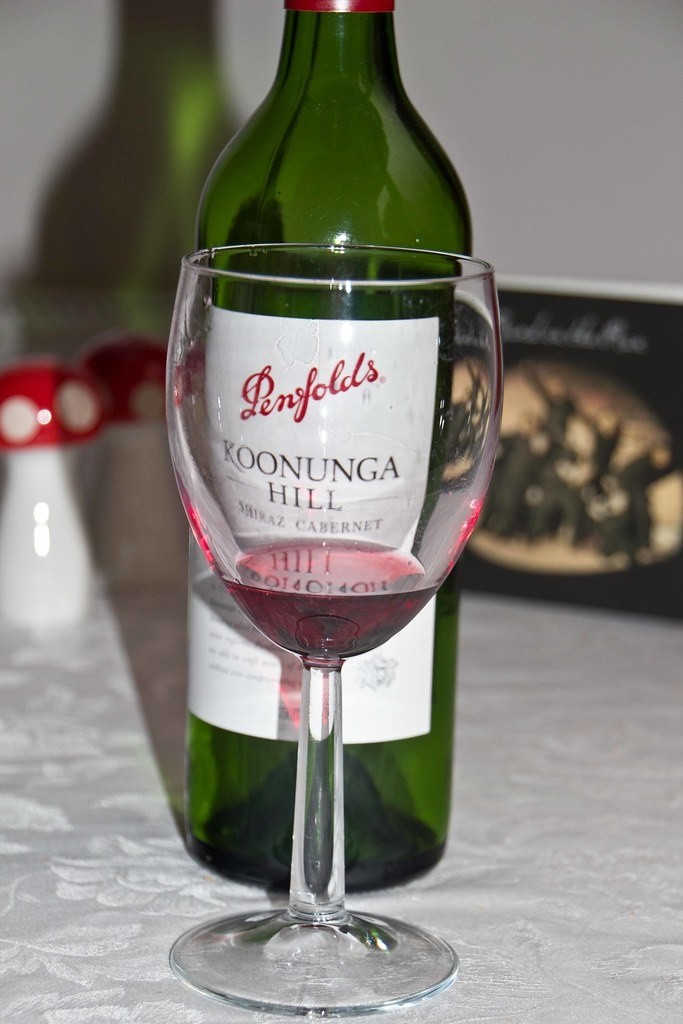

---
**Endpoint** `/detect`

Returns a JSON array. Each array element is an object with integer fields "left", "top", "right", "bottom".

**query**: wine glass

[{"left": 160, "top": 241, "right": 508, "bottom": 1018}]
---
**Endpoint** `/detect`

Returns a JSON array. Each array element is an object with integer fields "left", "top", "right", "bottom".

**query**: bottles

[{"left": 172, "top": 0, "right": 480, "bottom": 900}]
